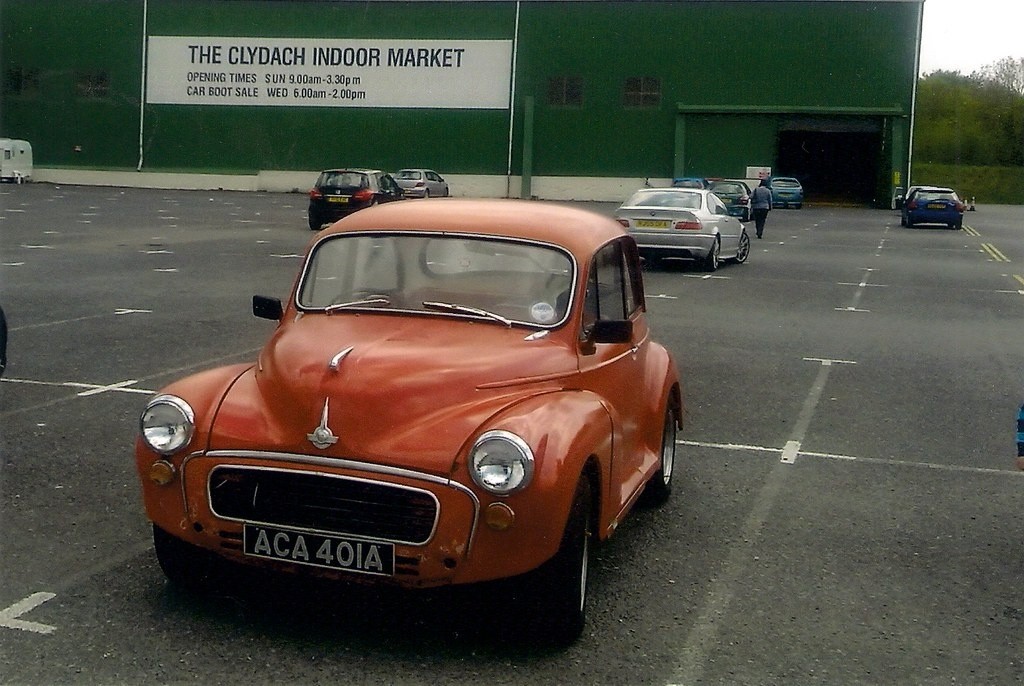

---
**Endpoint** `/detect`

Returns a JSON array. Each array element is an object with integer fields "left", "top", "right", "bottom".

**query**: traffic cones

[
  {"left": 963, "top": 196, "right": 968, "bottom": 212},
  {"left": 968, "top": 194, "right": 976, "bottom": 211}
]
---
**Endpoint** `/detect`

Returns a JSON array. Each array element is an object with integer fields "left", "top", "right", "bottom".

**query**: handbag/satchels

[{"left": 750, "top": 188, "right": 757, "bottom": 204}]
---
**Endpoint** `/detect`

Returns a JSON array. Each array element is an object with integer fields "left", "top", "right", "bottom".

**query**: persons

[
  {"left": 1016, "top": 405, "right": 1024, "bottom": 471},
  {"left": 750, "top": 179, "right": 772, "bottom": 239}
]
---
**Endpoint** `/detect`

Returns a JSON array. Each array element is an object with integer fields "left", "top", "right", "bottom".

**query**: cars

[
  {"left": 706, "top": 180, "right": 754, "bottom": 222},
  {"left": 900, "top": 186, "right": 964, "bottom": 230},
  {"left": 672, "top": 177, "right": 709, "bottom": 190},
  {"left": 133, "top": 199, "right": 684, "bottom": 644},
  {"left": 761, "top": 176, "right": 803, "bottom": 209},
  {"left": 611, "top": 187, "right": 750, "bottom": 272},
  {"left": 392, "top": 168, "right": 450, "bottom": 199},
  {"left": 308, "top": 168, "right": 406, "bottom": 230}
]
